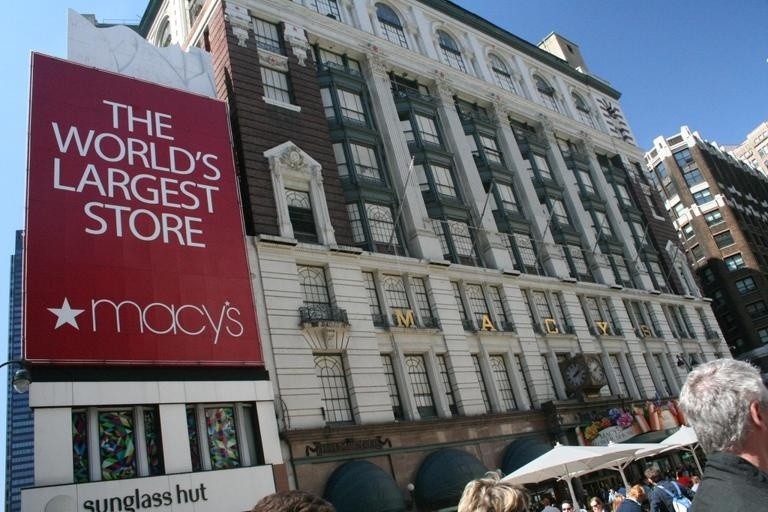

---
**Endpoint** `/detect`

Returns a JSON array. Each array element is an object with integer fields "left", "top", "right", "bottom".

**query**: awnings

[
  {"left": 414, "top": 448, "right": 491, "bottom": 510},
  {"left": 498, "top": 436, "right": 562, "bottom": 489},
  {"left": 322, "top": 460, "right": 407, "bottom": 510}
]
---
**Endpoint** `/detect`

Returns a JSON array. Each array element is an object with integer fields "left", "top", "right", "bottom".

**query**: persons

[
  {"left": 254, "top": 489, "right": 335, "bottom": 510},
  {"left": 677, "top": 358, "right": 766, "bottom": 511},
  {"left": 458, "top": 471, "right": 532, "bottom": 510},
  {"left": 532, "top": 462, "right": 706, "bottom": 510}
]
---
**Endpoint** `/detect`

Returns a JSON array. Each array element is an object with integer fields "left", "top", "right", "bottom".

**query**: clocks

[
  {"left": 587, "top": 356, "right": 604, "bottom": 381},
  {"left": 565, "top": 363, "right": 586, "bottom": 387}
]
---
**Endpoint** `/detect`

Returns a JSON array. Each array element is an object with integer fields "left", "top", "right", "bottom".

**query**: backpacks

[{"left": 672, "top": 494, "right": 692, "bottom": 512}]
